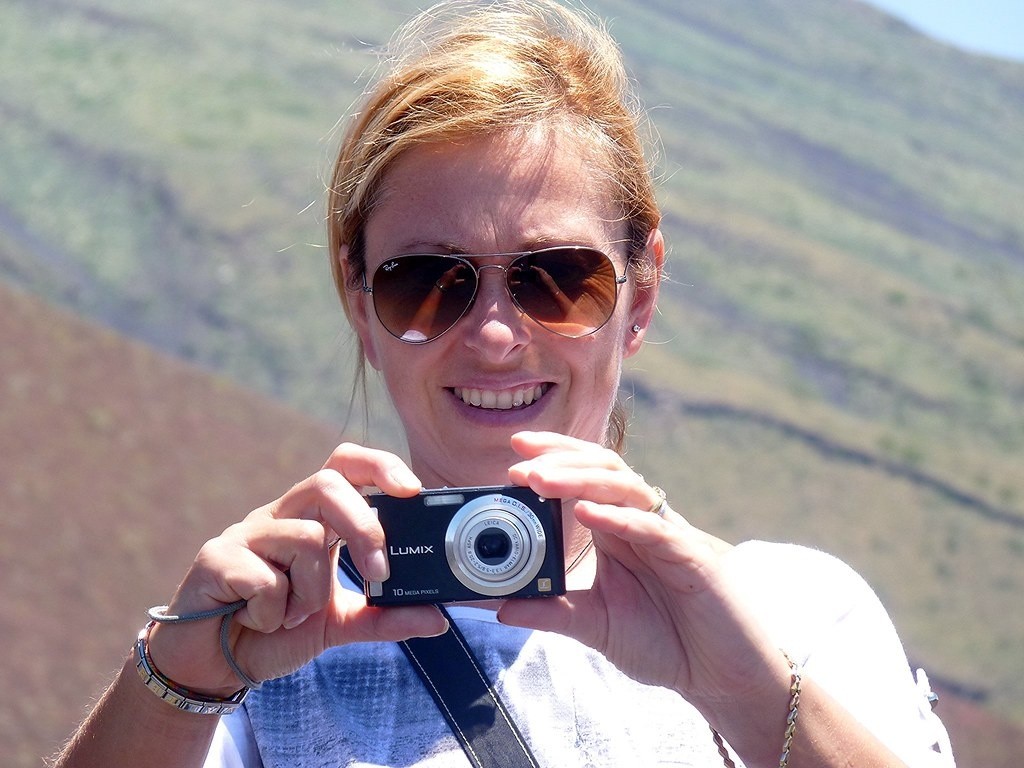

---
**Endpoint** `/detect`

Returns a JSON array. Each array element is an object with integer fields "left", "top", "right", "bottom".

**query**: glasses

[{"left": 354, "top": 246, "right": 636, "bottom": 347}]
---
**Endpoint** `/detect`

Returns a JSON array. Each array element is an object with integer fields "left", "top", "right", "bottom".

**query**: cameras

[{"left": 364, "top": 484, "right": 567, "bottom": 607}]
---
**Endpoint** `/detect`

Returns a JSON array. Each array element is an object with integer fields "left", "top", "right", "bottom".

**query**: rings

[{"left": 649, "top": 486, "right": 666, "bottom": 516}]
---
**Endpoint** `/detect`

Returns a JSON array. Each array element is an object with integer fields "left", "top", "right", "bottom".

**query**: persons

[{"left": 52, "top": 0, "right": 955, "bottom": 767}]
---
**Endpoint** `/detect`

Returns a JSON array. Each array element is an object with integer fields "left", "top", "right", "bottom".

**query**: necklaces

[{"left": 565, "top": 540, "right": 593, "bottom": 572}]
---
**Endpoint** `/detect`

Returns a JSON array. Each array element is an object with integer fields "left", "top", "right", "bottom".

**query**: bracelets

[
  {"left": 135, "top": 621, "right": 249, "bottom": 715},
  {"left": 709, "top": 648, "right": 801, "bottom": 768}
]
